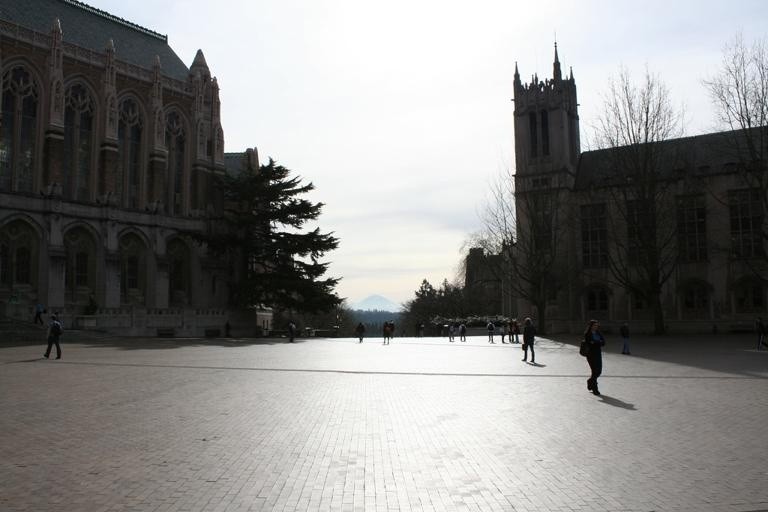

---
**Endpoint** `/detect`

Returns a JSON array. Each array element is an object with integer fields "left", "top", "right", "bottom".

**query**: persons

[
  {"left": 43, "top": 314, "right": 64, "bottom": 360},
  {"left": 354, "top": 323, "right": 366, "bottom": 343},
  {"left": 383, "top": 320, "right": 396, "bottom": 345},
  {"left": 619, "top": 319, "right": 631, "bottom": 354},
  {"left": 753, "top": 316, "right": 765, "bottom": 351},
  {"left": 35, "top": 300, "right": 44, "bottom": 325},
  {"left": 581, "top": 319, "right": 606, "bottom": 396},
  {"left": 522, "top": 317, "right": 535, "bottom": 362},
  {"left": 289, "top": 319, "right": 295, "bottom": 342},
  {"left": 447, "top": 321, "right": 467, "bottom": 342},
  {"left": 487, "top": 317, "right": 520, "bottom": 344}
]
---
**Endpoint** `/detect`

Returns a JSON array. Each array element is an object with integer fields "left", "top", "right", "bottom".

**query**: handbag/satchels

[
  {"left": 521, "top": 344, "right": 528, "bottom": 350},
  {"left": 578, "top": 334, "right": 592, "bottom": 357}
]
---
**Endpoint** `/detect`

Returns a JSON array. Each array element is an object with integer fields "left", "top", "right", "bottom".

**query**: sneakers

[
  {"left": 41, "top": 355, "right": 48, "bottom": 359},
  {"left": 587, "top": 378, "right": 600, "bottom": 395}
]
